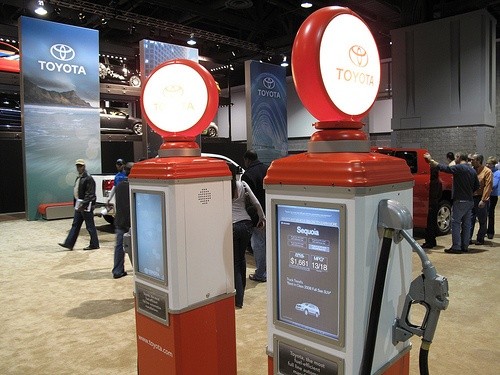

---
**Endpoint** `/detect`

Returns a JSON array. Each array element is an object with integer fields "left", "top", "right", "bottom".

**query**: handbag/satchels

[{"left": 243, "top": 183, "right": 263, "bottom": 227}]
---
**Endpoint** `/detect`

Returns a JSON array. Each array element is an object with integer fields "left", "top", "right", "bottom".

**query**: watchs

[{"left": 429, "top": 159, "right": 434, "bottom": 162}]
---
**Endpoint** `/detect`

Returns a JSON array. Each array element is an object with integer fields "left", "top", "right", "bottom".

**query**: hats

[
  {"left": 117, "top": 159, "right": 124, "bottom": 164},
  {"left": 75, "top": 159, "right": 85, "bottom": 165}
]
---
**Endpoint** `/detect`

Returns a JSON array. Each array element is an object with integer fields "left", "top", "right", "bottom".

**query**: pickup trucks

[{"left": 88, "top": 152, "right": 245, "bottom": 224}]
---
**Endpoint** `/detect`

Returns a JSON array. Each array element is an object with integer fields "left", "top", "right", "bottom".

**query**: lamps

[
  {"left": 34, "top": 0, "right": 47, "bottom": 15},
  {"left": 281, "top": 56, "right": 290, "bottom": 67},
  {"left": 186, "top": 35, "right": 197, "bottom": 46}
]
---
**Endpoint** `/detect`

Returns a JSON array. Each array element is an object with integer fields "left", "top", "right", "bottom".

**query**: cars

[
  {"left": 100, "top": 106, "right": 143, "bottom": 136},
  {"left": 0, "top": 41, "right": 20, "bottom": 73},
  {"left": 370, "top": 147, "right": 454, "bottom": 236},
  {"left": 201, "top": 122, "right": 219, "bottom": 138},
  {"left": 99, "top": 56, "right": 142, "bottom": 87},
  {"left": 0, "top": 95, "right": 21, "bottom": 129}
]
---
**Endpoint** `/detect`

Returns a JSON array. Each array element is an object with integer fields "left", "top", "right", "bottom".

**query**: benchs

[{"left": 38, "top": 202, "right": 75, "bottom": 220}]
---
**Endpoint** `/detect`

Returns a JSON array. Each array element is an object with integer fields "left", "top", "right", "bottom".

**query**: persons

[
  {"left": 470, "top": 155, "right": 493, "bottom": 245},
  {"left": 106, "top": 159, "right": 128, "bottom": 217},
  {"left": 240, "top": 150, "right": 269, "bottom": 282},
  {"left": 58, "top": 159, "right": 100, "bottom": 250},
  {"left": 111, "top": 163, "right": 134, "bottom": 278},
  {"left": 467, "top": 153, "right": 473, "bottom": 164},
  {"left": 420, "top": 164, "right": 443, "bottom": 248},
  {"left": 226, "top": 163, "right": 266, "bottom": 308},
  {"left": 485, "top": 158, "right": 500, "bottom": 239},
  {"left": 445, "top": 151, "right": 455, "bottom": 167},
  {"left": 423, "top": 152, "right": 480, "bottom": 254}
]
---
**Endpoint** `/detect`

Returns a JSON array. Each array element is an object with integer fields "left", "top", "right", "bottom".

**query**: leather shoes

[
  {"left": 475, "top": 241, "right": 484, "bottom": 245},
  {"left": 249, "top": 274, "right": 266, "bottom": 281},
  {"left": 420, "top": 243, "right": 436, "bottom": 248},
  {"left": 444, "top": 248, "right": 461, "bottom": 254}
]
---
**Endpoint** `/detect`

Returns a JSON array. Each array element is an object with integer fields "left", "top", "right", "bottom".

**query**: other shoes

[
  {"left": 114, "top": 271, "right": 127, "bottom": 278},
  {"left": 58, "top": 243, "right": 73, "bottom": 250},
  {"left": 487, "top": 234, "right": 493, "bottom": 238},
  {"left": 83, "top": 246, "right": 99, "bottom": 250}
]
